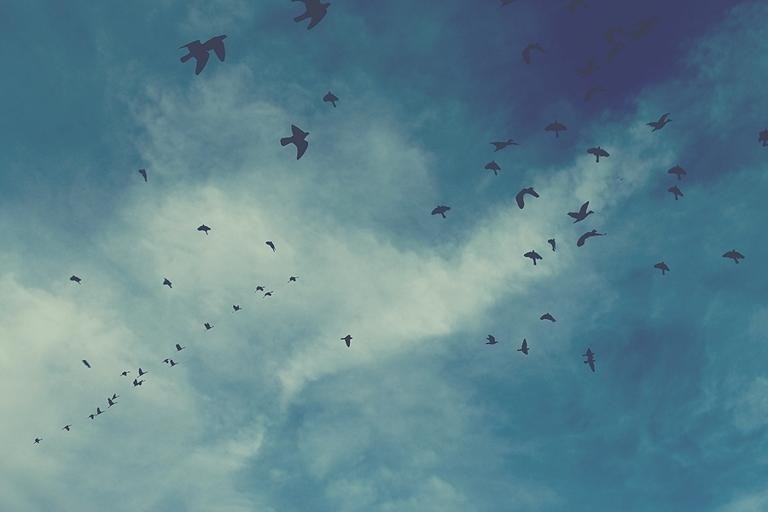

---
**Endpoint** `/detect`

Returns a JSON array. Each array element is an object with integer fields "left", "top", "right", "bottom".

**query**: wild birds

[
  {"left": 515, "top": 14, "right": 649, "bottom": 78},
  {"left": 667, "top": 185, "right": 685, "bottom": 201},
  {"left": 484, "top": 111, "right": 691, "bottom": 180},
  {"left": 759, "top": 128, "right": 768, "bottom": 147},
  {"left": 654, "top": 262, "right": 672, "bottom": 277},
  {"left": 265, "top": 240, "right": 280, "bottom": 252},
  {"left": 34, "top": 268, "right": 301, "bottom": 446},
  {"left": 483, "top": 311, "right": 602, "bottom": 375},
  {"left": 324, "top": 91, "right": 338, "bottom": 108},
  {"left": 197, "top": 224, "right": 213, "bottom": 237},
  {"left": 341, "top": 335, "right": 352, "bottom": 347},
  {"left": 292, "top": 0, "right": 330, "bottom": 30},
  {"left": 432, "top": 206, "right": 450, "bottom": 218},
  {"left": 138, "top": 169, "right": 147, "bottom": 182},
  {"left": 178, "top": 35, "right": 226, "bottom": 74},
  {"left": 281, "top": 125, "right": 309, "bottom": 160},
  {"left": 722, "top": 250, "right": 745, "bottom": 263},
  {"left": 514, "top": 186, "right": 609, "bottom": 267}
]
